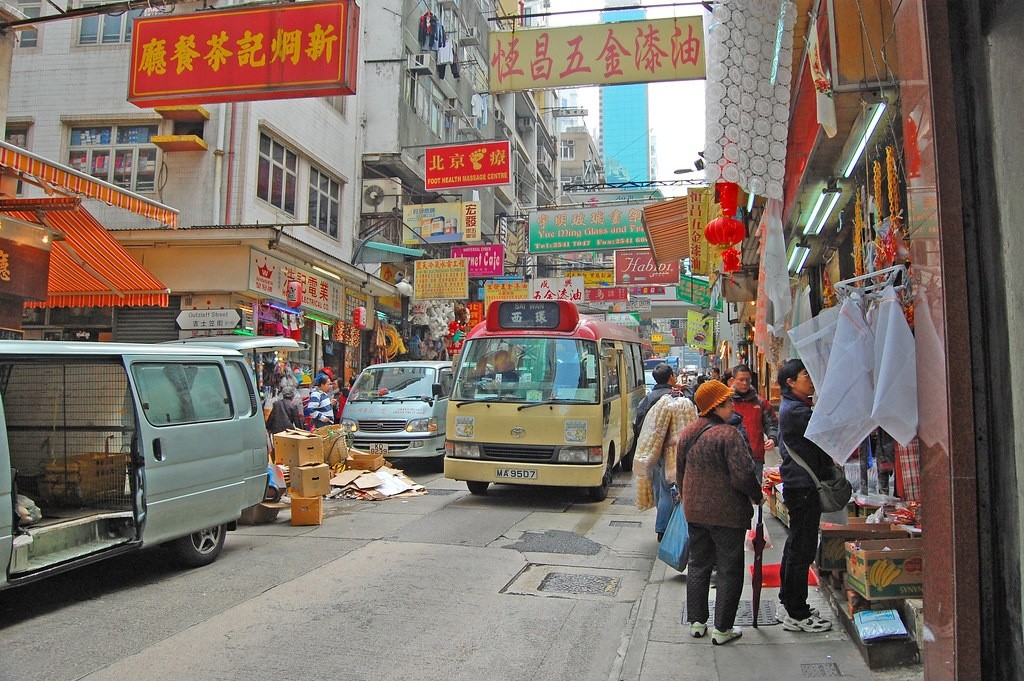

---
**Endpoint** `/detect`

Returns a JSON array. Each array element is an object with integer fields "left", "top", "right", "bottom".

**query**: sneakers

[
  {"left": 711, "top": 627, "right": 742, "bottom": 644},
  {"left": 782, "top": 614, "right": 832, "bottom": 632},
  {"left": 775, "top": 601, "right": 820, "bottom": 622},
  {"left": 689, "top": 622, "right": 707, "bottom": 637}
]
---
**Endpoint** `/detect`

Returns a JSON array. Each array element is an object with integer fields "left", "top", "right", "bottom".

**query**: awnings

[{"left": 0, "top": 196, "right": 170, "bottom": 309}]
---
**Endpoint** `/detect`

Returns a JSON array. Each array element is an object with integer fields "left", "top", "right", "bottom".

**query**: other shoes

[
  {"left": 658, "top": 533, "right": 663, "bottom": 542},
  {"left": 710, "top": 582, "right": 717, "bottom": 588}
]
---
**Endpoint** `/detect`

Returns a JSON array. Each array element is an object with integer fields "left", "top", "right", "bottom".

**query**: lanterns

[
  {"left": 287, "top": 279, "right": 302, "bottom": 310},
  {"left": 723, "top": 247, "right": 742, "bottom": 285},
  {"left": 705, "top": 217, "right": 747, "bottom": 248},
  {"left": 354, "top": 306, "right": 366, "bottom": 330}
]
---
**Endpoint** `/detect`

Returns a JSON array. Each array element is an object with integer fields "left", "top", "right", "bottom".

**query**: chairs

[
  {"left": 553, "top": 361, "right": 588, "bottom": 388},
  {"left": 380, "top": 375, "right": 399, "bottom": 395}
]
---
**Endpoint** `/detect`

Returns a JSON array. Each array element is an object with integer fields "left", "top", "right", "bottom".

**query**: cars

[{"left": 644, "top": 369, "right": 659, "bottom": 396}]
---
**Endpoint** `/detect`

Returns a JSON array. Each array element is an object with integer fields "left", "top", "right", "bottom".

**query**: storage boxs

[
  {"left": 288, "top": 463, "right": 334, "bottom": 498},
  {"left": 272, "top": 427, "right": 327, "bottom": 466},
  {"left": 753, "top": 468, "right": 927, "bottom": 598},
  {"left": 38, "top": 451, "right": 126, "bottom": 507},
  {"left": 348, "top": 454, "right": 386, "bottom": 472},
  {"left": 291, "top": 494, "right": 323, "bottom": 525}
]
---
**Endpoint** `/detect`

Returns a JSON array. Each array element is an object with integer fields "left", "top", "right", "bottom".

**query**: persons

[
  {"left": 308, "top": 373, "right": 337, "bottom": 428},
  {"left": 778, "top": 359, "right": 833, "bottom": 633},
  {"left": 479, "top": 350, "right": 519, "bottom": 395},
  {"left": 266, "top": 386, "right": 302, "bottom": 434},
  {"left": 681, "top": 373, "right": 691, "bottom": 385},
  {"left": 632, "top": 361, "right": 687, "bottom": 442},
  {"left": 729, "top": 365, "right": 778, "bottom": 485},
  {"left": 330, "top": 377, "right": 356, "bottom": 424},
  {"left": 712, "top": 368, "right": 720, "bottom": 381},
  {"left": 676, "top": 379, "right": 767, "bottom": 645},
  {"left": 691, "top": 372, "right": 705, "bottom": 392}
]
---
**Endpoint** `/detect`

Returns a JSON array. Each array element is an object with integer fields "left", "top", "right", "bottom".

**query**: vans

[
  {"left": 337, "top": 360, "right": 494, "bottom": 475},
  {"left": 643, "top": 355, "right": 699, "bottom": 389},
  {"left": 0, "top": 334, "right": 315, "bottom": 593}
]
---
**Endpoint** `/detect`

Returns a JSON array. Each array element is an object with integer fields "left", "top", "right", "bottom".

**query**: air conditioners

[
  {"left": 460, "top": 118, "right": 482, "bottom": 133},
  {"left": 494, "top": 109, "right": 506, "bottom": 122},
  {"left": 361, "top": 176, "right": 403, "bottom": 213},
  {"left": 567, "top": 139, "right": 574, "bottom": 145},
  {"left": 524, "top": 117, "right": 534, "bottom": 132},
  {"left": 574, "top": 176, "right": 582, "bottom": 182},
  {"left": 462, "top": 27, "right": 481, "bottom": 47},
  {"left": 407, "top": 53, "right": 437, "bottom": 75},
  {"left": 598, "top": 167, "right": 605, "bottom": 173},
  {"left": 536, "top": 147, "right": 553, "bottom": 177}
]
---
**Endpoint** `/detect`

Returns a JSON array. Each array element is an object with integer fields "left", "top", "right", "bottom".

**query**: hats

[
  {"left": 283, "top": 387, "right": 294, "bottom": 397},
  {"left": 693, "top": 380, "right": 735, "bottom": 416}
]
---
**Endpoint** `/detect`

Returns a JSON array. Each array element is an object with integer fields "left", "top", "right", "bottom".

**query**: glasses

[
  {"left": 797, "top": 373, "right": 808, "bottom": 379},
  {"left": 738, "top": 378, "right": 752, "bottom": 384}
]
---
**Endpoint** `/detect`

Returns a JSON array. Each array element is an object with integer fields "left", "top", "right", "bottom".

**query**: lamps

[
  {"left": 844, "top": 98, "right": 893, "bottom": 179},
  {"left": 787, "top": 244, "right": 813, "bottom": 275},
  {"left": 703, "top": 317, "right": 719, "bottom": 331},
  {"left": 801, "top": 188, "right": 845, "bottom": 237}
]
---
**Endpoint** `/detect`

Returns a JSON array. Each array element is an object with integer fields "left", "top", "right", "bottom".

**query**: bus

[{"left": 431, "top": 299, "right": 653, "bottom": 502}]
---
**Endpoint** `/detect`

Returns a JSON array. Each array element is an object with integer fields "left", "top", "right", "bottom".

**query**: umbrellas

[{"left": 752, "top": 505, "right": 766, "bottom": 629}]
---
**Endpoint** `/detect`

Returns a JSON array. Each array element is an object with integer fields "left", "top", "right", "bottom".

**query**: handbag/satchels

[
  {"left": 744, "top": 505, "right": 773, "bottom": 551},
  {"left": 657, "top": 484, "right": 690, "bottom": 572},
  {"left": 819, "top": 466, "right": 852, "bottom": 513}
]
